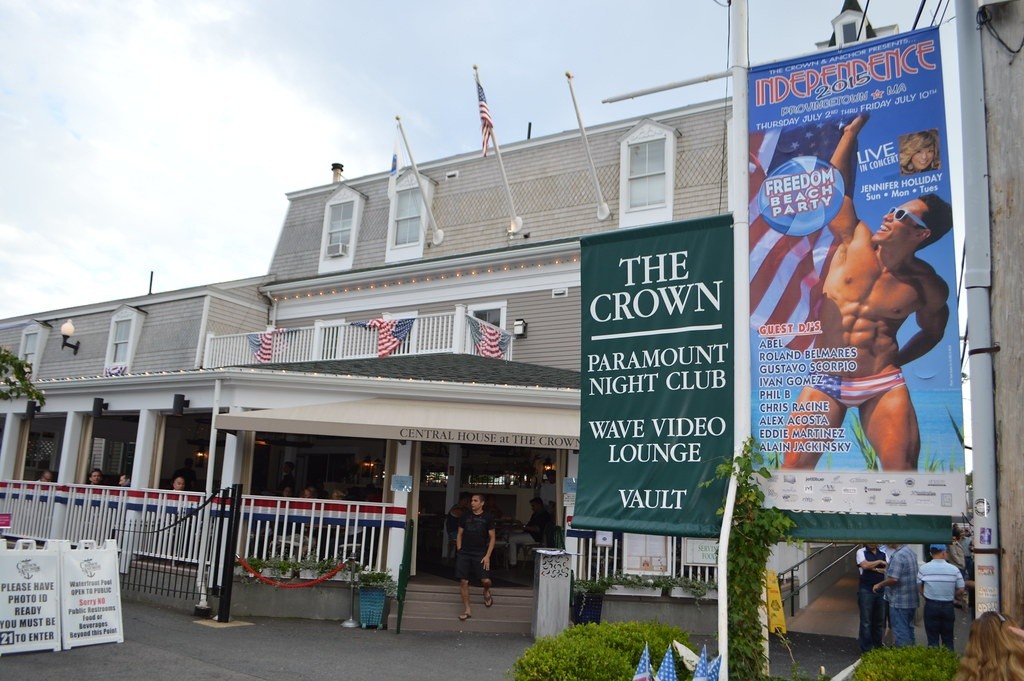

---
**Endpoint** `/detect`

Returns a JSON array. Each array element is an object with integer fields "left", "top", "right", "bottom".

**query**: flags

[
  {"left": 387, "top": 124, "right": 406, "bottom": 200},
  {"left": 474, "top": 75, "right": 494, "bottom": 157},
  {"left": 572, "top": 214, "right": 734, "bottom": 538},
  {"left": 631, "top": 644, "right": 723, "bottom": 681},
  {"left": 749, "top": 117, "right": 858, "bottom": 353}
]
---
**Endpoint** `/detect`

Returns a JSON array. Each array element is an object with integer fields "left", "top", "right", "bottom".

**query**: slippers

[
  {"left": 458, "top": 614, "right": 472, "bottom": 620},
  {"left": 483, "top": 591, "right": 493, "bottom": 607}
]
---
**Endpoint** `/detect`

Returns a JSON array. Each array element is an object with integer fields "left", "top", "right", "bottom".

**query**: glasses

[
  {"left": 889, "top": 207, "right": 927, "bottom": 228},
  {"left": 43, "top": 476, "right": 53, "bottom": 481}
]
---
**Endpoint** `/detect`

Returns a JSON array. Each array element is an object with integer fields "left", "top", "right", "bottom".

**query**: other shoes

[{"left": 509, "top": 565, "right": 517, "bottom": 569}]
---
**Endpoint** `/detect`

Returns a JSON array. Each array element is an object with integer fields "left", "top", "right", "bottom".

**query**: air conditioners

[{"left": 326, "top": 242, "right": 346, "bottom": 258}]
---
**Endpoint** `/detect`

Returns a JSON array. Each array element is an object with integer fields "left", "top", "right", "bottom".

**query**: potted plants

[
  {"left": 668, "top": 577, "right": 718, "bottom": 600},
  {"left": 358, "top": 571, "right": 396, "bottom": 629},
  {"left": 572, "top": 579, "right": 604, "bottom": 625},
  {"left": 319, "top": 557, "right": 352, "bottom": 581},
  {"left": 606, "top": 574, "right": 668, "bottom": 597},
  {"left": 298, "top": 558, "right": 320, "bottom": 579},
  {"left": 235, "top": 557, "right": 266, "bottom": 578},
  {"left": 266, "top": 558, "right": 296, "bottom": 579}
]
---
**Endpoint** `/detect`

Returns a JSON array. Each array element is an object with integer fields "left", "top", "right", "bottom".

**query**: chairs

[{"left": 444, "top": 519, "right": 548, "bottom": 571}]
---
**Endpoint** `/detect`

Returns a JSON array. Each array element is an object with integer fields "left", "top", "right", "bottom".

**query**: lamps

[
  {"left": 26, "top": 400, "right": 40, "bottom": 418},
  {"left": 61, "top": 319, "right": 80, "bottom": 354},
  {"left": 173, "top": 393, "right": 190, "bottom": 415},
  {"left": 515, "top": 319, "right": 528, "bottom": 338},
  {"left": 92, "top": 397, "right": 108, "bottom": 417}
]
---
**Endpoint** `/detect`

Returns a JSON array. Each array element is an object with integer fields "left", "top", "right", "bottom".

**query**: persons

[
  {"left": 505, "top": 468, "right": 557, "bottom": 568},
  {"left": 278, "top": 461, "right": 383, "bottom": 544},
  {"left": 782, "top": 110, "right": 953, "bottom": 474},
  {"left": 447, "top": 492, "right": 471, "bottom": 541},
  {"left": 89, "top": 469, "right": 131, "bottom": 487},
  {"left": 36, "top": 471, "right": 54, "bottom": 482},
  {"left": 856, "top": 543, "right": 965, "bottom": 655},
  {"left": 899, "top": 129, "right": 939, "bottom": 175},
  {"left": 456, "top": 494, "right": 496, "bottom": 621},
  {"left": 171, "top": 457, "right": 196, "bottom": 491},
  {"left": 949, "top": 524, "right": 973, "bottom": 581},
  {"left": 954, "top": 611, "right": 1024, "bottom": 681}
]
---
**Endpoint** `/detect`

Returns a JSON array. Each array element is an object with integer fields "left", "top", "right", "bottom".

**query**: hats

[{"left": 930, "top": 544, "right": 947, "bottom": 551}]
